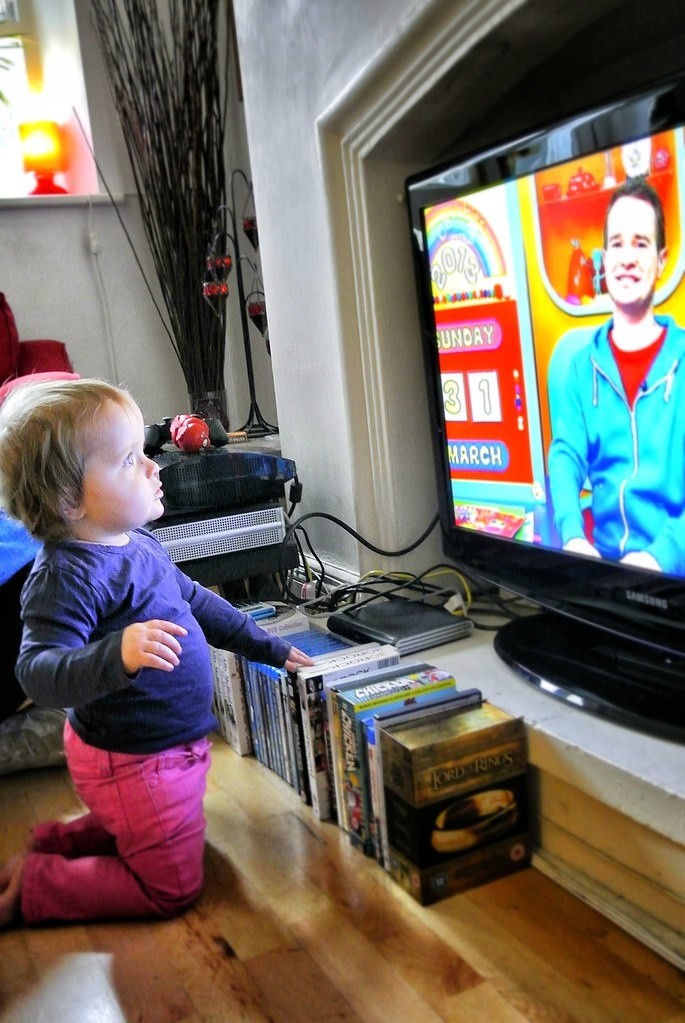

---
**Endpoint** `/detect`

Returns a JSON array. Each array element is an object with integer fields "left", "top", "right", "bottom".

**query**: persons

[
  {"left": 548, "top": 177, "right": 685, "bottom": 578},
  {"left": 0, "top": 378, "right": 314, "bottom": 926}
]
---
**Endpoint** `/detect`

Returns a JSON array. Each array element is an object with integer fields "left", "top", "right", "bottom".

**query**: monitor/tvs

[{"left": 401, "top": 72, "right": 685, "bottom": 745}]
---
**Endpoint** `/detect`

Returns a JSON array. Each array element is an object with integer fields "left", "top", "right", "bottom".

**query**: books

[{"left": 208, "top": 600, "right": 532, "bottom": 907}]
